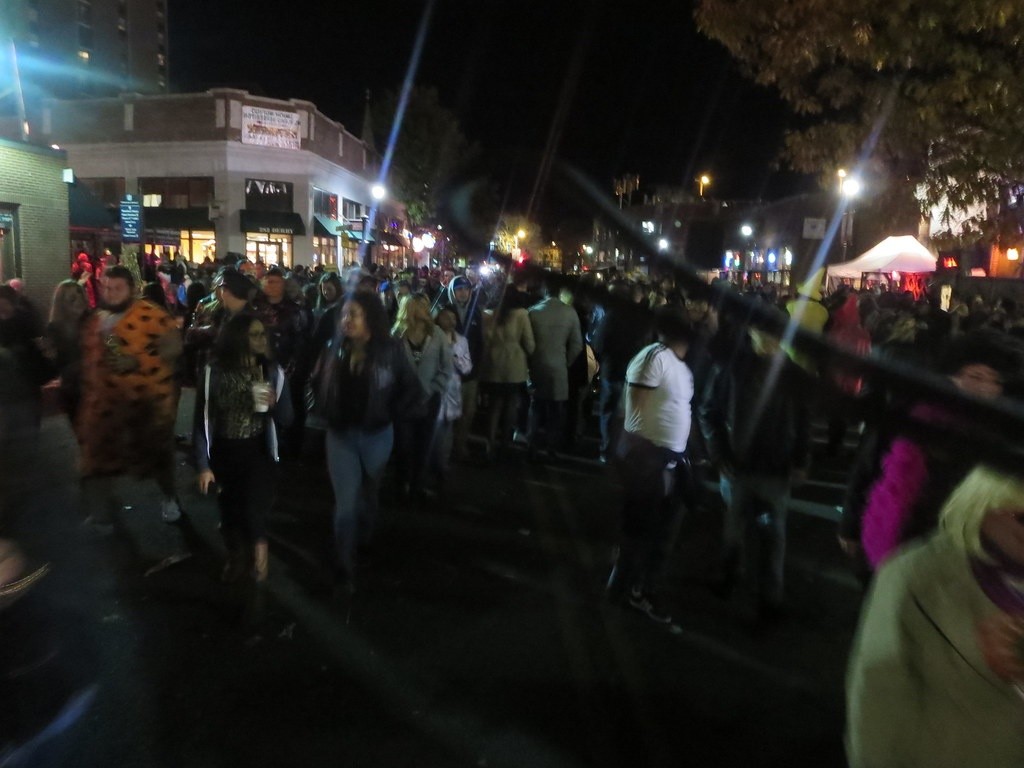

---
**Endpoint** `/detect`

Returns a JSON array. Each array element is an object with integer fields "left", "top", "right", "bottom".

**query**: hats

[
  {"left": 454, "top": 277, "right": 471, "bottom": 289},
  {"left": 213, "top": 264, "right": 258, "bottom": 295}
]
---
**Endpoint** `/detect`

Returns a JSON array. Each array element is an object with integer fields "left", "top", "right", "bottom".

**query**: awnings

[
  {"left": 68, "top": 207, "right": 214, "bottom": 231},
  {"left": 314, "top": 216, "right": 353, "bottom": 238},
  {"left": 348, "top": 230, "right": 375, "bottom": 242},
  {"left": 239, "top": 209, "right": 306, "bottom": 236}
]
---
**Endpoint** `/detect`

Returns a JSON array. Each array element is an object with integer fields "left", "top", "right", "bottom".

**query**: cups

[{"left": 253, "top": 383, "right": 270, "bottom": 413}]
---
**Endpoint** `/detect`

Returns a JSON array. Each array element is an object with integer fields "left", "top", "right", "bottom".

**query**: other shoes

[
  {"left": 72, "top": 515, "right": 114, "bottom": 542},
  {"left": 512, "top": 430, "right": 527, "bottom": 443},
  {"left": 253, "top": 556, "right": 269, "bottom": 583},
  {"left": 221, "top": 553, "right": 241, "bottom": 584},
  {"left": 606, "top": 575, "right": 632, "bottom": 593},
  {"left": 628, "top": 584, "right": 672, "bottom": 622},
  {"left": 159, "top": 482, "right": 189, "bottom": 521}
]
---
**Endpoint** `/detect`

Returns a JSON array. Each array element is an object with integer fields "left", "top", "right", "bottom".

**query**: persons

[
  {"left": 698, "top": 301, "right": 830, "bottom": 600},
  {"left": 845, "top": 462, "right": 1024, "bottom": 768},
  {"left": 0, "top": 247, "right": 1024, "bottom": 768},
  {"left": 860, "top": 338, "right": 1024, "bottom": 567},
  {"left": 191, "top": 311, "right": 294, "bottom": 583},
  {"left": 305, "top": 289, "right": 425, "bottom": 594},
  {"left": 607, "top": 305, "right": 695, "bottom": 623}
]
